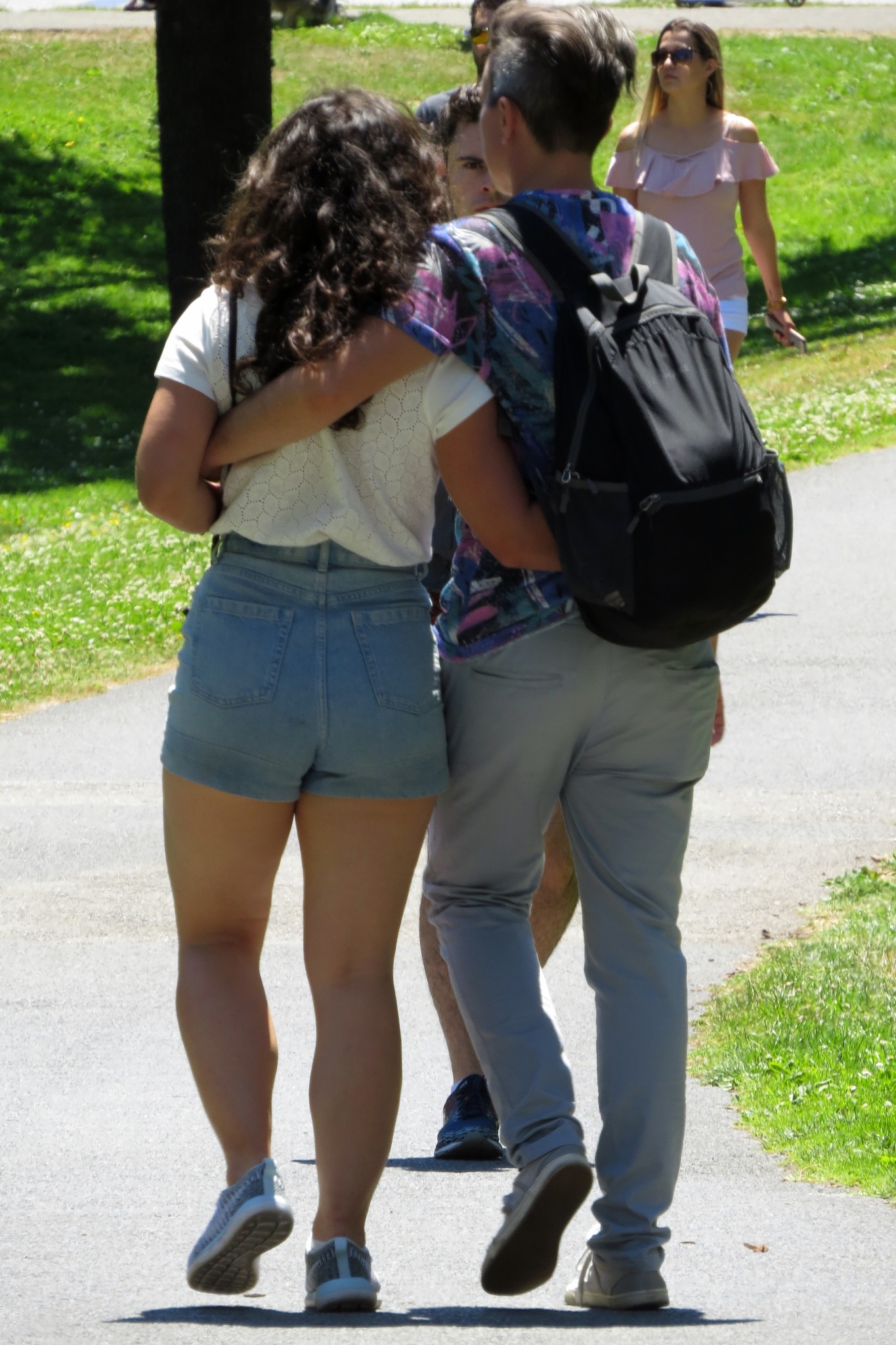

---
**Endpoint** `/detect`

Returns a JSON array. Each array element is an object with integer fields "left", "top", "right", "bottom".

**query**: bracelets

[{"left": 767, "top": 298, "right": 787, "bottom": 309}]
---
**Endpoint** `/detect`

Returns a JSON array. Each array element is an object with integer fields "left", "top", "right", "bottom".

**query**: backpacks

[{"left": 494, "top": 203, "right": 792, "bottom": 648}]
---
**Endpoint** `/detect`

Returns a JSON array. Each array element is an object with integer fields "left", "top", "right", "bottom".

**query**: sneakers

[
  {"left": 304, "top": 1237, "right": 380, "bottom": 1310},
  {"left": 434, "top": 1071, "right": 502, "bottom": 1158},
  {"left": 187, "top": 1158, "right": 294, "bottom": 1295},
  {"left": 480, "top": 1143, "right": 593, "bottom": 1297},
  {"left": 562, "top": 1247, "right": 668, "bottom": 1308}
]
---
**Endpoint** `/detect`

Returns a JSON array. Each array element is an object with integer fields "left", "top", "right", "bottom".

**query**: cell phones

[{"left": 763, "top": 314, "right": 807, "bottom": 353}]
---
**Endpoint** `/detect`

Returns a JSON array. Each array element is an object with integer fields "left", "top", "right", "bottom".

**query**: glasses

[
  {"left": 651, "top": 48, "right": 700, "bottom": 62},
  {"left": 462, "top": 26, "right": 489, "bottom": 42}
]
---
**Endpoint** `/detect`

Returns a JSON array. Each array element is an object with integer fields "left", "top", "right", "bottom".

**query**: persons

[
  {"left": 606, "top": 20, "right": 796, "bottom": 361},
  {"left": 413, "top": 0, "right": 506, "bottom": 154},
  {"left": 418, "top": 89, "right": 580, "bottom": 1157},
  {"left": 208, "top": 0, "right": 728, "bottom": 1307},
  {"left": 134, "top": 92, "right": 559, "bottom": 1315}
]
